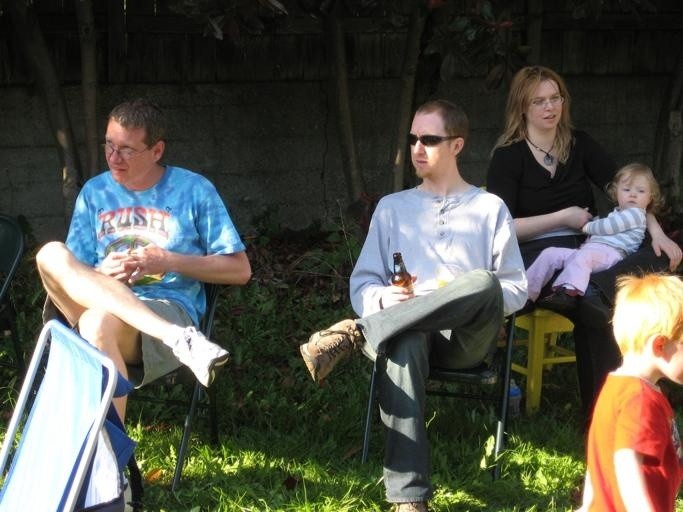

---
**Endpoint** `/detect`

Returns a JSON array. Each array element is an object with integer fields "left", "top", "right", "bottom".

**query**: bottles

[
  {"left": 390, "top": 251, "right": 414, "bottom": 298},
  {"left": 508, "top": 378, "right": 523, "bottom": 418}
]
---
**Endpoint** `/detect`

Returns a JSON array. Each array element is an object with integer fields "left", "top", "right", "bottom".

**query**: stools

[{"left": 483, "top": 307, "right": 575, "bottom": 416}]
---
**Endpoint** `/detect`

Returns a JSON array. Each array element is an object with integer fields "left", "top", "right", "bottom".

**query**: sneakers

[
  {"left": 173, "top": 327, "right": 229, "bottom": 388},
  {"left": 299, "top": 318, "right": 364, "bottom": 386}
]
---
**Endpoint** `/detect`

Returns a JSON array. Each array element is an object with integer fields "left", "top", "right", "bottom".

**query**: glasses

[
  {"left": 101, "top": 143, "right": 151, "bottom": 159},
  {"left": 407, "top": 132, "right": 462, "bottom": 146},
  {"left": 525, "top": 96, "right": 565, "bottom": 106}
]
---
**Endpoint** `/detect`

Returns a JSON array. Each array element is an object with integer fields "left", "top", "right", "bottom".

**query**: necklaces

[{"left": 525, "top": 135, "right": 561, "bottom": 167}]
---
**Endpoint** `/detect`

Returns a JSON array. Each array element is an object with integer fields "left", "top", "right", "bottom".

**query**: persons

[
  {"left": 579, "top": 272, "right": 682, "bottom": 511},
  {"left": 516, "top": 161, "right": 662, "bottom": 301},
  {"left": 297, "top": 101, "right": 530, "bottom": 511},
  {"left": 33, "top": 99, "right": 253, "bottom": 436},
  {"left": 484, "top": 63, "right": 682, "bottom": 423}
]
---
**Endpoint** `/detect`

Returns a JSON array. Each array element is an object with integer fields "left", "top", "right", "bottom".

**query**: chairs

[
  {"left": 0, "top": 215, "right": 27, "bottom": 375},
  {"left": 360, "top": 312, "right": 516, "bottom": 481},
  {"left": 0, "top": 317, "right": 120, "bottom": 512},
  {"left": 127, "top": 285, "right": 220, "bottom": 493}
]
